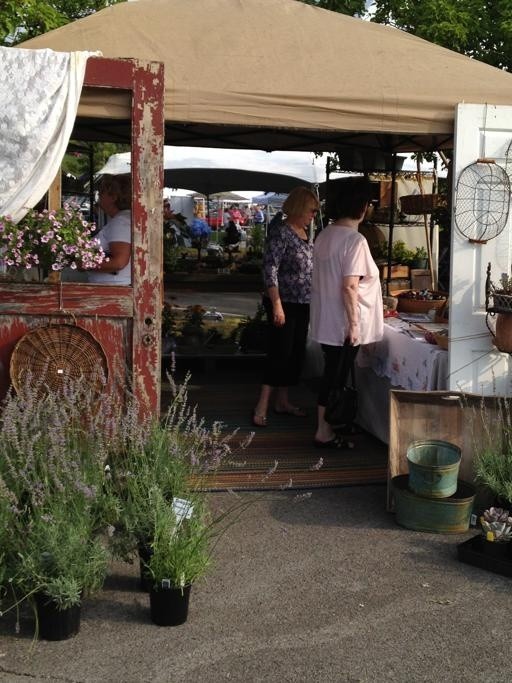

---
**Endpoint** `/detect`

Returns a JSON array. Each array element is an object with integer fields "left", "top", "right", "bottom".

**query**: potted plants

[
  {"left": 479, "top": 503, "right": 511, "bottom": 557},
  {"left": 413, "top": 244, "right": 430, "bottom": 269}
]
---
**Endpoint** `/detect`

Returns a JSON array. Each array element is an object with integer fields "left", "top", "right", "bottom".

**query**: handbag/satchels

[{"left": 323, "top": 384, "right": 359, "bottom": 425}]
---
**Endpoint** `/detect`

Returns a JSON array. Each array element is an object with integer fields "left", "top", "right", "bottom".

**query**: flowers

[
  {"left": 164, "top": 198, "right": 271, "bottom": 346},
  {"left": 0, "top": 351, "right": 326, "bottom": 660},
  {"left": 0, "top": 194, "right": 111, "bottom": 283}
]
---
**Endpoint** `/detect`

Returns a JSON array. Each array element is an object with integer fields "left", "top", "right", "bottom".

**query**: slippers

[
  {"left": 314, "top": 433, "right": 354, "bottom": 451},
  {"left": 276, "top": 403, "right": 308, "bottom": 417},
  {"left": 253, "top": 409, "right": 270, "bottom": 427}
]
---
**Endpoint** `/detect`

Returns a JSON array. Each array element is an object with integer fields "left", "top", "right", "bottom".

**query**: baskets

[
  {"left": 400, "top": 194, "right": 442, "bottom": 214},
  {"left": 453, "top": 159, "right": 511, "bottom": 244},
  {"left": 397, "top": 290, "right": 447, "bottom": 314}
]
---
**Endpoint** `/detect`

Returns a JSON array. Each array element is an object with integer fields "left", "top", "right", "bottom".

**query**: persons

[
  {"left": 310, "top": 173, "right": 386, "bottom": 451},
  {"left": 253, "top": 183, "right": 319, "bottom": 429},
  {"left": 221, "top": 200, "right": 267, "bottom": 228},
  {"left": 83, "top": 173, "right": 134, "bottom": 284}
]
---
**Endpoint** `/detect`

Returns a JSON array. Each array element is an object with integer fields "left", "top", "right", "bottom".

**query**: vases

[
  {"left": 47, "top": 268, "right": 62, "bottom": 285},
  {"left": 389, "top": 472, "right": 477, "bottom": 536}
]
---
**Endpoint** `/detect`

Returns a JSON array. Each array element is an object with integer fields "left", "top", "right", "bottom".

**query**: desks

[{"left": 355, "top": 313, "right": 449, "bottom": 448}]
